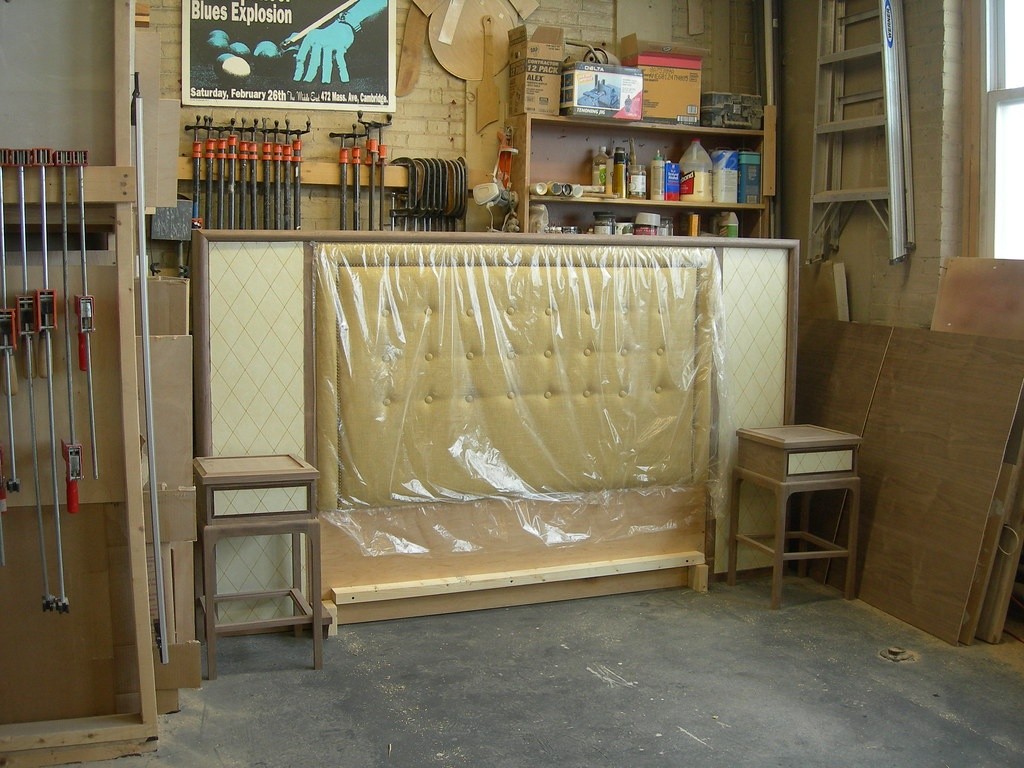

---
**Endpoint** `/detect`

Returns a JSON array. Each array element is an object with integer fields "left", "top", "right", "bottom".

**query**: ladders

[{"left": 806, "top": 0, "right": 916, "bottom": 264}]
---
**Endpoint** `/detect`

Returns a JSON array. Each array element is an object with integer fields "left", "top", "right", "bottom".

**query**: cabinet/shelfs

[{"left": 503, "top": 113, "right": 772, "bottom": 238}]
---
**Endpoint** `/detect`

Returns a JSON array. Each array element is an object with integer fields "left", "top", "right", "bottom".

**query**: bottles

[
  {"left": 544, "top": 211, "right": 739, "bottom": 238},
  {"left": 591, "top": 145, "right": 626, "bottom": 199},
  {"left": 650, "top": 137, "right": 712, "bottom": 201}
]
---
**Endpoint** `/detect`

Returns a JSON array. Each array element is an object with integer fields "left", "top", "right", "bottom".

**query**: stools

[
  {"left": 727, "top": 464, "right": 865, "bottom": 611},
  {"left": 196, "top": 451, "right": 325, "bottom": 682}
]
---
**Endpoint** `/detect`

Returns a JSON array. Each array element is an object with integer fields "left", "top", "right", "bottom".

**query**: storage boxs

[
  {"left": 700, "top": 90, "right": 764, "bottom": 129},
  {"left": 713, "top": 150, "right": 738, "bottom": 203},
  {"left": 120, "top": 273, "right": 201, "bottom": 717},
  {"left": 507, "top": 21, "right": 566, "bottom": 117},
  {"left": 561, "top": 61, "right": 645, "bottom": 123},
  {"left": 621, "top": 33, "right": 711, "bottom": 126},
  {"left": 739, "top": 150, "right": 761, "bottom": 203},
  {"left": 732, "top": 421, "right": 868, "bottom": 484}
]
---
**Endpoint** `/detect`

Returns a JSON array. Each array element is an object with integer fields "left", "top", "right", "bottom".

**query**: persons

[{"left": 280, "top": 0, "right": 389, "bottom": 83}]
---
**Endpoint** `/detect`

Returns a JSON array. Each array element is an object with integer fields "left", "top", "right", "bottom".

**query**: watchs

[{"left": 338, "top": 11, "right": 362, "bottom": 33}]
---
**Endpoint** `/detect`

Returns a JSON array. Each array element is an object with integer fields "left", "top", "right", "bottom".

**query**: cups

[{"left": 627, "top": 164, "right": 647, "bottom": 200}]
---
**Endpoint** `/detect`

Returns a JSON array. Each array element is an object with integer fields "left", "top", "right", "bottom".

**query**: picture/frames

[{"left": 181, "top": 0, "right": 397, "bottom": 113}]
